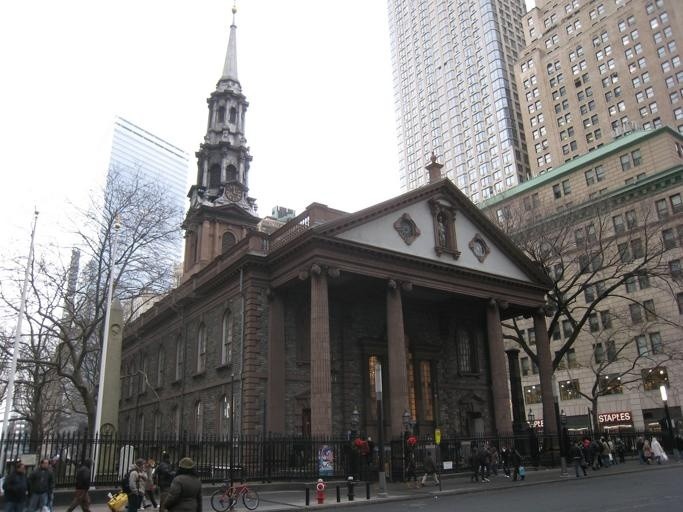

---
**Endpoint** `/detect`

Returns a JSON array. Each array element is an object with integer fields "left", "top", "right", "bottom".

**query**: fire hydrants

[
  {"left": 345, "top": 476, "right": 357, "bottom": 501},
  {"left": 313, "top": 478, "right": 328, "bottom": 504}
]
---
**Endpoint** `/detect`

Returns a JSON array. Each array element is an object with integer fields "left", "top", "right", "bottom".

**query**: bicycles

[{"left": 210, "top": 474, "right": 260, "bottom": 511}]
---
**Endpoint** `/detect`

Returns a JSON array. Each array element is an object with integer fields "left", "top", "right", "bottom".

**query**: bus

[
  {"left": 563, "top": 424, "right": 633, "bottom": 437},
  {"left": 646, "top": 421, "right": 660, "bottom": 434}
]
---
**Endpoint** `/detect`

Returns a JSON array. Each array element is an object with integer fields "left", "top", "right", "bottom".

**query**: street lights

[
  {"left": 559, "top": 408, "right": 567, "bottom": 429},
  {"left": 401, "top": 409, "right": 413, "bottom": 477},
  {"left": 658, "top": 381, "right": 677, "bottom": 454},
  {"left": 349, "top": 405, "right": 360, "bottom": 479}
]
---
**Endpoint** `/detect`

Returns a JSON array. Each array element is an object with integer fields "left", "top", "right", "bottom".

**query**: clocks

[{"left": 225, "top": 182, "right": 242, "bottom": 203}]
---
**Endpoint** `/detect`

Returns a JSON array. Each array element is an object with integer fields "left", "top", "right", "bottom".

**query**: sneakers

[
  {"left": 139, "top": 505, "right": 162, "bottom": 511},
  {"left": 481, "top": 474, "right": 512, "bottom": 484}
]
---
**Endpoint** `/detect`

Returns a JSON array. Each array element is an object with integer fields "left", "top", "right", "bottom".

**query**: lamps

[
  {"left": 400, "top": 406, "right": 411, "bottom": 431},
  {"left": 559, "top": 408, "right": 568, "bottom": 426},
  {"left": 349, "top": 403, "right": 360, "bottom": 430}
]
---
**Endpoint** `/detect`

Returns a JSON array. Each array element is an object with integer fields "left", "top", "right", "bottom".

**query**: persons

[
  {"left": 0, "top": 450, "right": 203, "bottom": 512},
  {"left": 636, "top": 435, "right": 683, "bottom": 465},
  {"left": 405, "top": 449, "right": 419, "bottom": 488},
  {"left": 467, "top": 445, "right": 524, "bottom": 483},
  {"left": 421, "top": 451, "right": 439, "bottom": 487},
  {"left": 569, "top": 435, "right": 625, "bottom": 477}
]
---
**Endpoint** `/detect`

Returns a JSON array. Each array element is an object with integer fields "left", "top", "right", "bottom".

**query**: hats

[
  {"left": 176, "top": 455, "right": 196, "bottom": 469},
  {"left": 134, "top": 457, "right": 146, "bottom": 466}
]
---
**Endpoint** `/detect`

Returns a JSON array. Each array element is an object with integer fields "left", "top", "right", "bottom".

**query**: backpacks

[{"left": 121, "top": 468, "right": 139, "bottom": 493}]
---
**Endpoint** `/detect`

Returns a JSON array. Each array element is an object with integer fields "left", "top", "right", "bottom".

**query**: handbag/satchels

[{"left": 518, "top": 466, "right": 526, "bottom": 476}]
